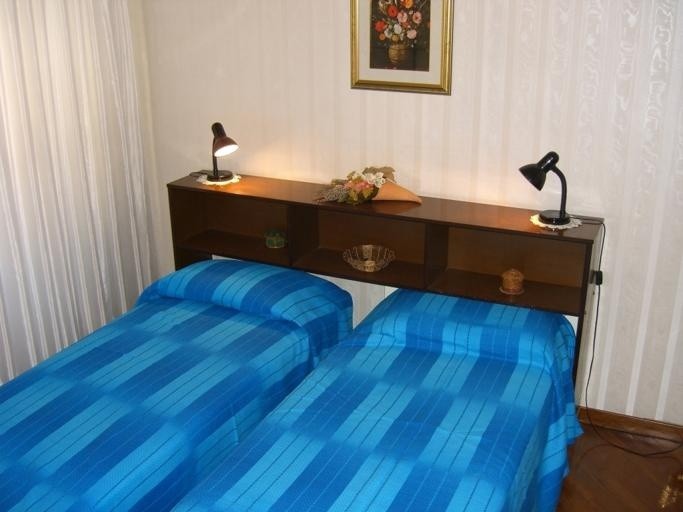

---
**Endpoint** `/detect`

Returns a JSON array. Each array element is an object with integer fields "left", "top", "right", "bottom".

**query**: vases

[{"left": 388, "top": 43, "right": 414, "bottom": 68}]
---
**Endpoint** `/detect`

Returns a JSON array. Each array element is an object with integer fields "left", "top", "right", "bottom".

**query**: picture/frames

[{"left": 347, "top": 0, "right": 455, "bottom": 94}]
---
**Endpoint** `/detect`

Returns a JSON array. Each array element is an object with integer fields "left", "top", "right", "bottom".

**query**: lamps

[
  {"left": 520, "top": 150, "right": 572, "bottom": 225},
  {"left": 202, "top": 121, "right": 239, "bottom": 182}
]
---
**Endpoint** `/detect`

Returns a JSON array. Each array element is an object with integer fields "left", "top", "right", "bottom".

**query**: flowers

[
  {"left": 372, "top": 0, "right": 429, "bottom": 48},
  {"left": 314, "top": 167, "right": 401, "bottom": 205}
]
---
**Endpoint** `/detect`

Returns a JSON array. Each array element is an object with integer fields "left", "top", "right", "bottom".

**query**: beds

[{"left": 0, "top": 259, "right": 576, "bottom": 509}]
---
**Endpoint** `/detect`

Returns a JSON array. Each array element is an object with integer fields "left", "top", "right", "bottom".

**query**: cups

[{"left": 502, "top": 269, "right": 524, "bottom": 292}]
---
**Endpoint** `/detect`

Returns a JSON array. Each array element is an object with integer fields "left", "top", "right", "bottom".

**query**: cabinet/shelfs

[{"left": 163, "top": 169, "right": 603, "bottom": 419}]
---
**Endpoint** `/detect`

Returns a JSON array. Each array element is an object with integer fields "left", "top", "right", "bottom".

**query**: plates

[{"left": 499, "top": 285, "right": 525, "bottom": 295}]
